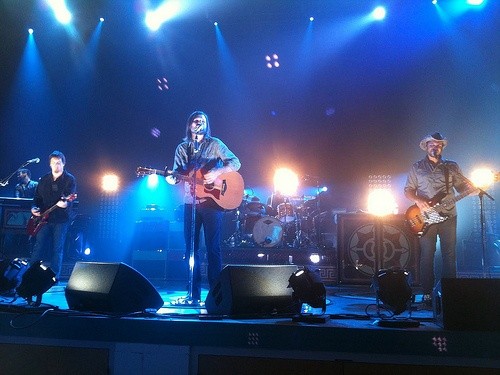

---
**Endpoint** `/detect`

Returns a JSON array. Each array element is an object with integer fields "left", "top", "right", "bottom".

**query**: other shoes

[{"left": 426, "top": 297, "right": 433, "bottom": 303}]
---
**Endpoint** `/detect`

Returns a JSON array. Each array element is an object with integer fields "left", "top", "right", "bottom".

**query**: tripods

[{"left": 292, "top": 202, "right": 320, "bottom": 250}]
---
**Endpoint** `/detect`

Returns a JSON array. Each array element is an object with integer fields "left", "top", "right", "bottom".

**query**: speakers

[
  {"left": 337, "top": 214, "right": 422, "bottom": 286},
  {"left": 432, "top": 278, "right": 500, "bottom": 333},
  {"left": 204, "top": 263, "right": 299, "bottom": 316},
  {"left": 64, "top": 220, "right": 202, "bottom": 315}
]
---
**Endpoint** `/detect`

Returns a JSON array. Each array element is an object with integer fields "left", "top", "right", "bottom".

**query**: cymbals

[{"left": 291, "top": 196, "right": 316, "bottom": 201}]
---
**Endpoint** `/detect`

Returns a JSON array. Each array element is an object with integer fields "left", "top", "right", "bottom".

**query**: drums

[
  {"left": 297, "top": 207, "right": 311, "bottom": 221},
  {"left": 276, "top": 203, "right": 295, "bottom": 223},
  {"left": 241, "top": 215, "right": 263, "bottom": 236},
  {"left": 252, "top": 215, "right": 286, "bottom": 248},
  {"left": 246, "top": 202, "right": 266, "bottom": 218}
]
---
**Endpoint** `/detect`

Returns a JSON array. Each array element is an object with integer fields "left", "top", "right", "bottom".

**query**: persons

[
  {"left": 165, "top": 111, "right": 242, "bottom": 308},
  {"left": 26, "top": 150, "right": 77, "bottom": 286},
  {"left": 14, "top": 169, "right": 39, "bottom": 198},
  {"left": 266, "top": 184, "right": 289, "bottom": 209},
  {"left": 403, "top": 131, "right": 498, "bottom": 312}
]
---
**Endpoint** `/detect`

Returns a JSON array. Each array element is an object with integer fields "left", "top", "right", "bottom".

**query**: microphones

[
  {"left": 27, "top": 157, "right": 40, "bottom": 163},
  {"left": 431, "top": 151, "right": 438, "bottom": 160},
  {"left": 194, "top": 123, "right": 203, "bottom": 132}
]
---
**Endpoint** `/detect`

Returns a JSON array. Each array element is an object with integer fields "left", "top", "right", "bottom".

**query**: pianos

[{"left": 2, "top": 197, "right": 83, "bottom": 260}]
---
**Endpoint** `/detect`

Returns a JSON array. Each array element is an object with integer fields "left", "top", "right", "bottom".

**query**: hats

[{"left": 419, "top": 132, "right": 449, "bottom": 151}]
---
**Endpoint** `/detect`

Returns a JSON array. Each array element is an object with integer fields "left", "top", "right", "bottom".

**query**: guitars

[
  {"left": 403, "top": 171, "right": 500, "bottom": 239},
  {"left": 26, "top": 192, "right": 78, "bottom": 237},
  {"left": 135, "top": 165, "right": 245, "bottom": 213}
]
